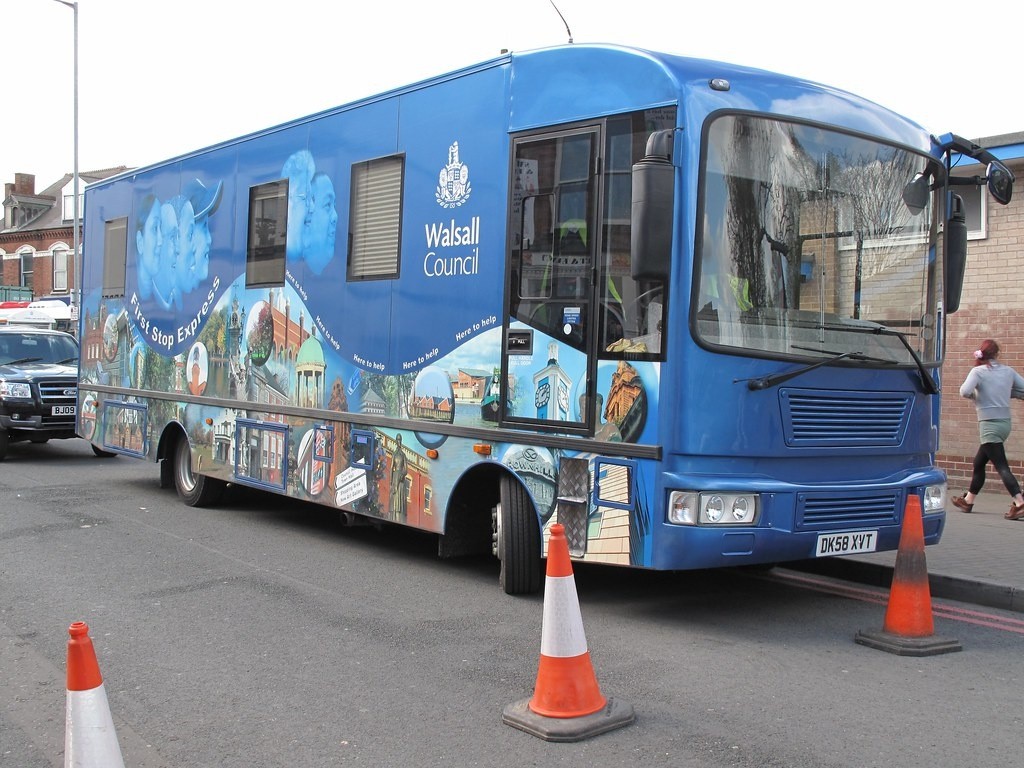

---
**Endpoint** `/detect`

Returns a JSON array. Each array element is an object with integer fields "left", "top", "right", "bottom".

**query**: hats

[{"left": 180, "top": 177, "right": 224, "bottom": 221}]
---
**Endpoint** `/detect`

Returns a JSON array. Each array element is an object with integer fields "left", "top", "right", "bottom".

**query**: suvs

[{"left": 0, "top": 322, "right": 118, "bottom": 459}]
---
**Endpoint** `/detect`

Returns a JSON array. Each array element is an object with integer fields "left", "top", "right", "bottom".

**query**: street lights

[{"left": 55, "top": 1, "right": 81, "bottom": 318}]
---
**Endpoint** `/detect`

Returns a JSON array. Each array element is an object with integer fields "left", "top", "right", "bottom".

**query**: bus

[{"left": 76, "top": 48, "right": 1017, "bottom": 606}]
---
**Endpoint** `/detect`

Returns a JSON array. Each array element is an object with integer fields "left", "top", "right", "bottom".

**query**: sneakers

[
  {"left": 1004, "top": 501, "right": 1024, "bottom": 519},
  {"left": 951, "top": 492, "right": 974, "bottom": 513}
]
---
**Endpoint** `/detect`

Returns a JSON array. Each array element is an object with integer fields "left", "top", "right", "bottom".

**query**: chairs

[{"left": 538, "top": 214, "right": 627, "bottom": 354}]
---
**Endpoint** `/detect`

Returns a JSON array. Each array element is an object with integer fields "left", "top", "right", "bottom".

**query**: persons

[
  {"left": 699, "top": 272, "right": 757, "bottom": 320},
  {"left": 951, "top": 339, "right": 1024, "bottom": 519}
]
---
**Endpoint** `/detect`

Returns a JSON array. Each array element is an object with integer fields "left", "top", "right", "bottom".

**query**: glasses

[{"left": 289, "top": 192, "right": 316, "bottom": 207}]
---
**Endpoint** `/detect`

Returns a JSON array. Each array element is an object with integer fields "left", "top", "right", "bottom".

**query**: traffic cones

[
  {"left": 498, "top": 522, "right": 634, "bottom": 744},
  {"left": 56, "top": 617, "right": 136, "bottom": 768},
  {"left": 854, "top": 499, "right": 963, "bottom": 659}
]
